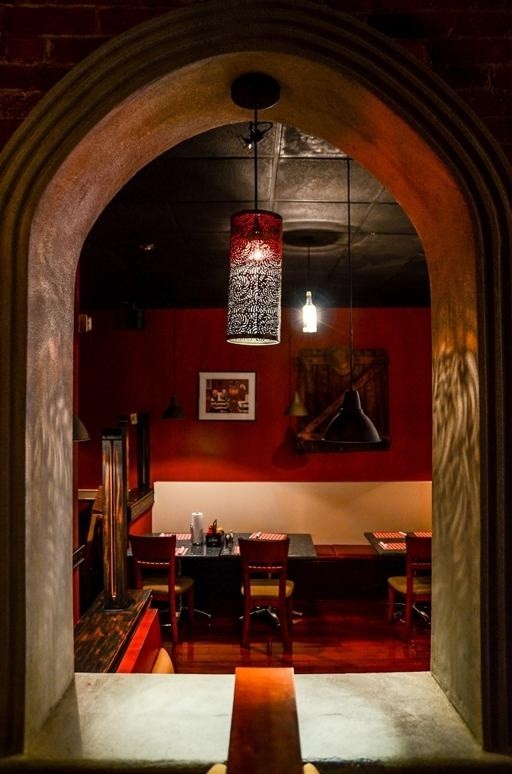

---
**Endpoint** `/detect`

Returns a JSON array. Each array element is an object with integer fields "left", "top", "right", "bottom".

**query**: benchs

[{"left": 153, "top": 481, "right": 432, "bottom": 561}]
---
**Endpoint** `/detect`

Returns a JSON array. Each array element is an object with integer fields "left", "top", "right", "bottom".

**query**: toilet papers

[{"left": 191, "top": 513, "right": 204, "bottom": 545}]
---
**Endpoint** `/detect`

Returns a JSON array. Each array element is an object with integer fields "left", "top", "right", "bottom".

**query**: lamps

[
  {"left": 322, "top": 156, "right": 383, "bottom": 444},
  {"left": 283, "top": 390, "right": 309, "bottom": 417},
  {"left": 300, "top": 228, "right": 318, "bottom": 334},
  {"left": 225, "top": 70, "right": 284, "bottom": 347},
  {"left": 74, "top": 415, "right": 91, "bottom": 442},
  {"left": 160, "top": 153, "right": 188, "bottom": 419}
]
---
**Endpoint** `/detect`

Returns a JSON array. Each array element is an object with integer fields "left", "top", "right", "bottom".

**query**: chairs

[
  {"left": 239, "top": 537, "right": 296, "bottom": 645},
  {"left": 385, "top": 535, "right": 432, "bottom": 640},
  {"left": 126, "top": 533, "right": 197, "bottom": 642}
]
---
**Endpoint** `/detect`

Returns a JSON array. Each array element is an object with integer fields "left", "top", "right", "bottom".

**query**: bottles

[{"left": 226, "top": 531, "right": 234, "bottom": 548}]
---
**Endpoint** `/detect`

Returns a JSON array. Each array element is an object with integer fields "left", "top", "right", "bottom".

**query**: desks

[
  {"left": 156, "top": 532, "right": 224, "bottom": 628},
  {"left": 364, "top": 531, "right": 432, "bottom": 628},
  {"left": 220, "top": 534, "right": 318, "bottom": 629}
]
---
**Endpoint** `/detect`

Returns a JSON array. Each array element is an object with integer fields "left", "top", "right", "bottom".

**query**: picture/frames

[{"left": 198, "top": 371, "right": 256, "bottom": 421}]
